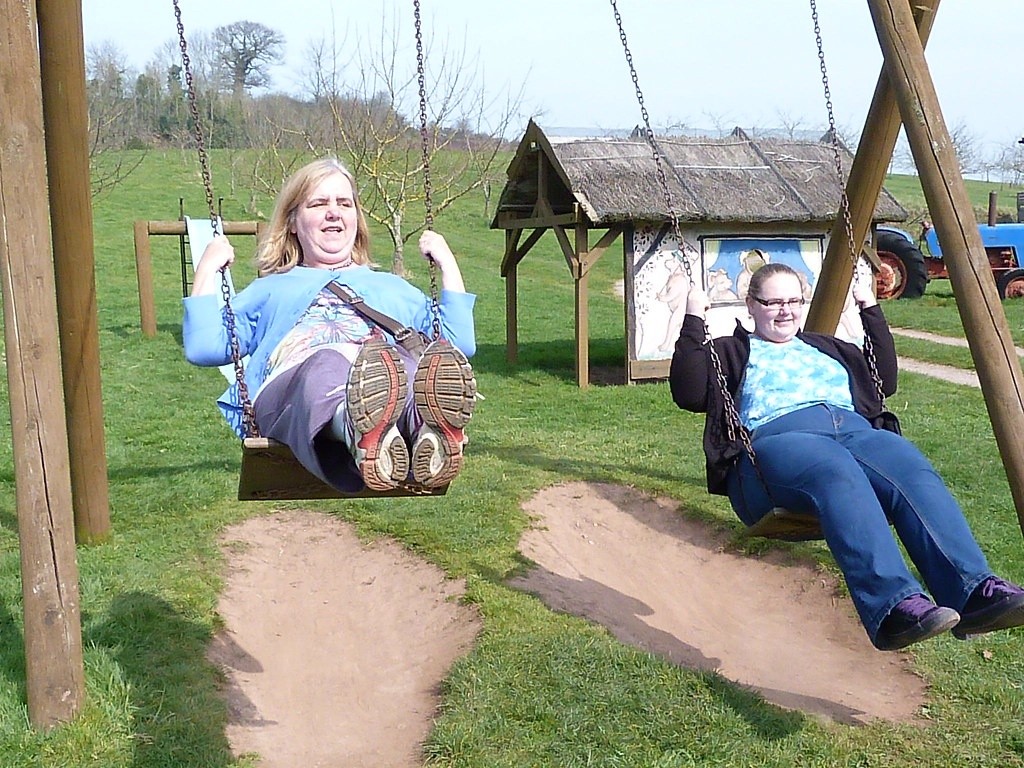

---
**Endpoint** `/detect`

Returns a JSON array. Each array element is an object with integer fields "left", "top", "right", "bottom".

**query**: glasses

[{"left": 748, "top": 293, "right": 805, "bottom": 306}]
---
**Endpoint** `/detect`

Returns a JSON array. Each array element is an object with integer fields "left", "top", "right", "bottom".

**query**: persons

[
  {"left": 668, "top": 263, "right": 1024, "bottom": 652},
  {"left": 180, "top": 158, "right": 478, "bottom": 492}
]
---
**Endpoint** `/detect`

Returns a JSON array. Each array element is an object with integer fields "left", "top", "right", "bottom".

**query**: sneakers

[
  {"left": 950, "top": 574, "right": 1024, "bottom": 640},
  {"left": 874, "top": 594, "right": 961, "bottom": 651}
]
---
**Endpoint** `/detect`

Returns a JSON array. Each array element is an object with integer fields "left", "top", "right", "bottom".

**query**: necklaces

[{"left": 300, "top": 259, "right": 354, "bottom": 271}]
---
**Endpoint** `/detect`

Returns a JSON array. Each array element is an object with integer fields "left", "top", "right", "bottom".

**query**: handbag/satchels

[{"left": 397, "top": 328, "right": 434, "bottom": 362}]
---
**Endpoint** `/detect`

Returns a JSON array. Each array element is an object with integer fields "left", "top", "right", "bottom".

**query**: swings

[
  {"left": 608, "top": 0, "right": 892, "bottom": 538},
  {"left": 171, "top": 2, "right": 452, "bottom": 502}
]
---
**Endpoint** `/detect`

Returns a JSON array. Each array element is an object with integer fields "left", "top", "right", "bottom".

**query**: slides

[{"left": 184, "top": 218, "right": 251, "bottom": 386}]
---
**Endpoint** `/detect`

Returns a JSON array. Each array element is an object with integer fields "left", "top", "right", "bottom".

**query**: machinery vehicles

[{"left": 861, "top": 190, "right": 1024, "bottom": 303}]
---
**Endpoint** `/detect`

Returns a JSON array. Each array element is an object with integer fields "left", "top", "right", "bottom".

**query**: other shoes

[
  {"left": 346, "top": 336, "right": 410, "bottom": 491},
  {"left": 411, "top": 338, "right": 477, "bottom": 488}
]
